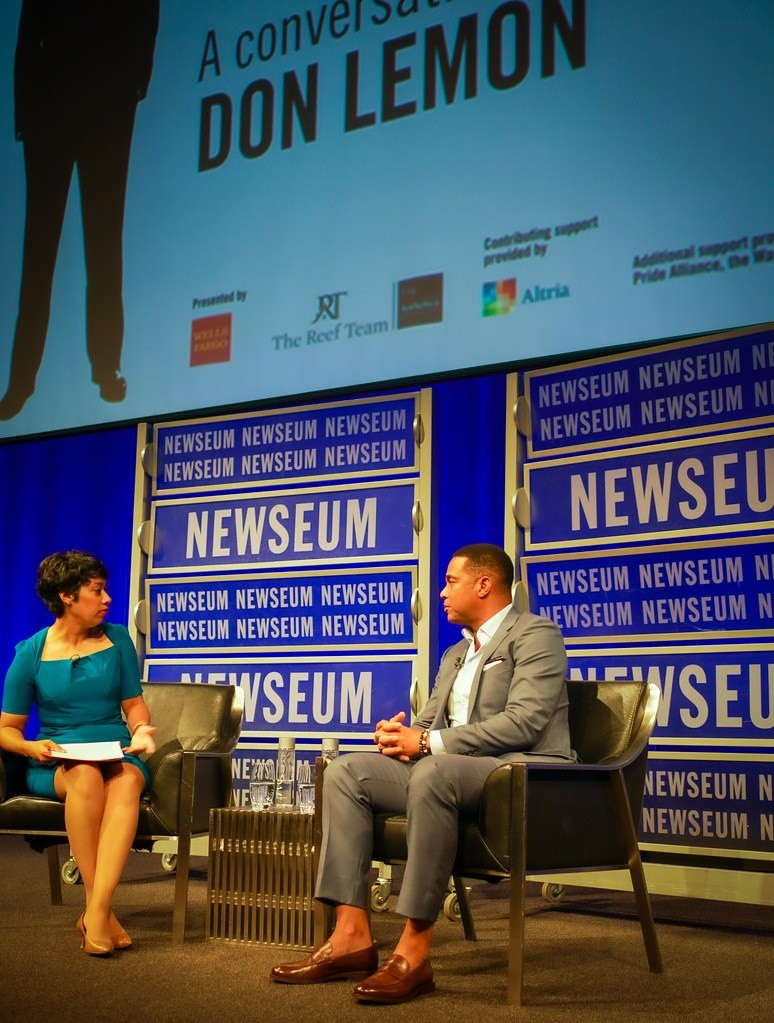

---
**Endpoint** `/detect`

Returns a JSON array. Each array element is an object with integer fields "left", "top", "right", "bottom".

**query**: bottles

[
  {"left": 321, "top": 737, "right": 339, "bottom": 771},
  {"left": 277, "top": 738, "right": 295, "bottom": 810}
]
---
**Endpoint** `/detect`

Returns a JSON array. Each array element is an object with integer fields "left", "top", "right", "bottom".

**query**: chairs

[
  {"left": 372, "top": 682, "right": 659, "bottom": 1007},
  {"left": 0, "top": 684, "right": 247, "bottom": 945}
]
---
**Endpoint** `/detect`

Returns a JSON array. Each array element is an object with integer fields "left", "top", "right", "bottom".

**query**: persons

[
  {"left": 0, "top": 550, "right": 157, "bottom": 954},
  {"left": 270, "top": 543, "right": 578, "bottom": 1003},
  {"left": 0, "top": 0, "right": 160, "bottom": 420}
]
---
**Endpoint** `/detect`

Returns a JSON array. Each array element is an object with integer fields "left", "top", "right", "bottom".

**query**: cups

[
  {"left": 248, "top": 761, "right": 275, "bottom": 811},
  {"left": 296, "top": 765, "right": 315, "bottom": 814}
]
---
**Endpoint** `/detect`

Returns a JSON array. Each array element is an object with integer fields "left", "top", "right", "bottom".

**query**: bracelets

[
  {"left": 132, "top": 724, "right": 142, "bottom": 735},
  {"left": 419, "top": 729, "right": 431, "bottom": 756}
]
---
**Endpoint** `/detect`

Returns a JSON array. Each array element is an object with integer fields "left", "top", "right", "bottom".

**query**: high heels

[
  {"left": 110, "top": 932, "right": 133, "bottom": 948},
  {"left": 76, "top": 911, "right": 115, "bottom": 953}
]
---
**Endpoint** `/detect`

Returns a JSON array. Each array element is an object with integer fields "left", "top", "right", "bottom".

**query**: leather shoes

[
  {"left": 270, "top": 939, "right": 379, "bottom": 984},
  {"left": 351, "top": 954, "right": 436, "bottom": 1003}
]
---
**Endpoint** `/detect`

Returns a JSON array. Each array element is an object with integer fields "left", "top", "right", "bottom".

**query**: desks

[{"left": 206, "top": 805, "right": 334, "bottom": 952}]
